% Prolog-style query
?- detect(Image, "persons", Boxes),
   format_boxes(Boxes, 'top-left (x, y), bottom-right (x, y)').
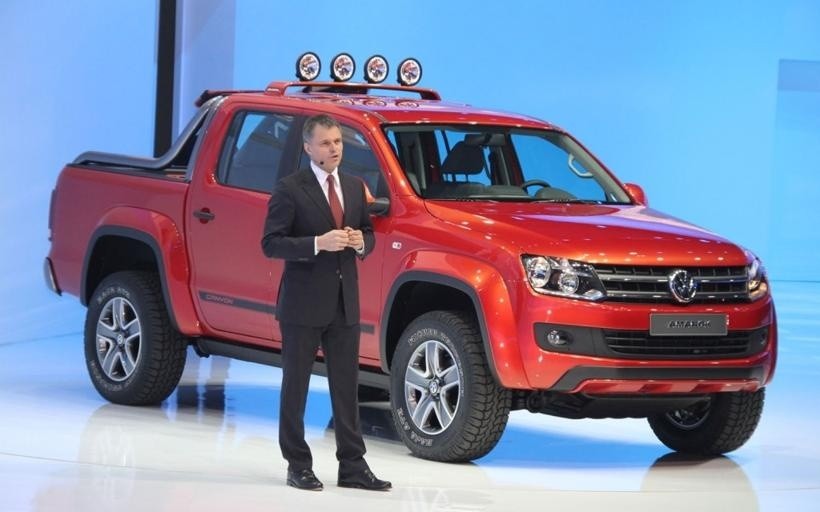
top-left (259, 112), bottom-right (396, 492)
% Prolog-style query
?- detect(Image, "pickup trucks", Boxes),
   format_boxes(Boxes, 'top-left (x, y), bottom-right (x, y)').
top-left (44, 50), bottom-right (782, 465)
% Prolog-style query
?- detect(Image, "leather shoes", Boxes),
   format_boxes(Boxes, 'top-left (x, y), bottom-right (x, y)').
top-left (287, 467), bottom-right (323, 491)
top-left (339, 467), bottom-right (391, 490)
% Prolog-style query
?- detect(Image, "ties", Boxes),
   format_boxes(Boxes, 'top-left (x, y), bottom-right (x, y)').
top-left (327, 174), bottom-right (344, 230)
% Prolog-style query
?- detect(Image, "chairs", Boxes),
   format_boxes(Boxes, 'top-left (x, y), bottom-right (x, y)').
top-left (422, 142), bottom-right (484, 199)
